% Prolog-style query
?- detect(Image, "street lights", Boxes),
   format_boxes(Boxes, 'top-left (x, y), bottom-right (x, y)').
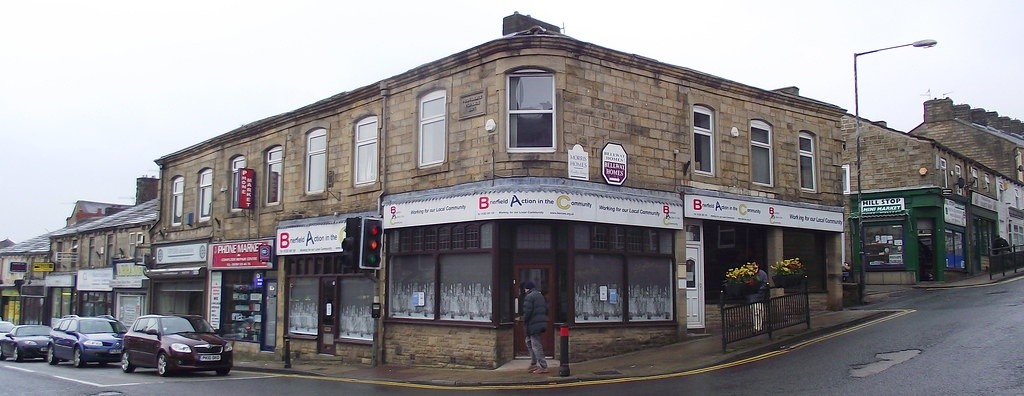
top-left (854, 39), bottom-right (939, 304)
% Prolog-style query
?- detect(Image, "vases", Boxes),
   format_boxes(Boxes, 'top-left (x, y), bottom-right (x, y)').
top-left (771, 273), bottom-right (803, 289)
top-left (724, 284), bottom-right (752, 300)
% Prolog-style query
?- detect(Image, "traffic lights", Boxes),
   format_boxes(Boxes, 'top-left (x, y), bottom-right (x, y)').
top-left (341, 218), bottom-right (361, 270)
top-left (359, 217), bottom-right (383, 270)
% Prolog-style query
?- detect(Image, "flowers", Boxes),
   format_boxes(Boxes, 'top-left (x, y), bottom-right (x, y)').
top-left (722, 262), bottom-right (760, 291)
top-left (768, 257), bottom-right (806, 275)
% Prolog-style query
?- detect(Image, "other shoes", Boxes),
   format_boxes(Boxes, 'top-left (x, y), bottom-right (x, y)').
top-left (528, 364), bottom-right (548, 373)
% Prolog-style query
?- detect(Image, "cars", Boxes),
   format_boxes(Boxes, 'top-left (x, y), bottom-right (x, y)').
top-left (0, 321), bottom-right (16, 338)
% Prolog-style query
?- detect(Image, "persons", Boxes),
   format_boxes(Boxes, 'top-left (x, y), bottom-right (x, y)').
top-left (992, 234), bottom-right (1011, 254)
top-left (749, 261), bottom-right (768, 333)
top-left (523, 281), bottom-right (548, 373)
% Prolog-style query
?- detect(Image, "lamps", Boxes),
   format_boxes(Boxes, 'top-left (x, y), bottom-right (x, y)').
top-left (954, 177), bottom-right (964, 187)
top-left (1017, 165), bottom-right (1024, 171)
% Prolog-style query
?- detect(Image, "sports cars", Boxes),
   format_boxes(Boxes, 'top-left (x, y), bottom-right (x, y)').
top-left (0, 325), bottom-right (53, 362)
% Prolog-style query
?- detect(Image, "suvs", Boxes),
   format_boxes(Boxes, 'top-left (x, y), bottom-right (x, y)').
top-left (121, 314), bottom-right (233, 377)
top-left (46, 314), bottom-right (128, 368)
